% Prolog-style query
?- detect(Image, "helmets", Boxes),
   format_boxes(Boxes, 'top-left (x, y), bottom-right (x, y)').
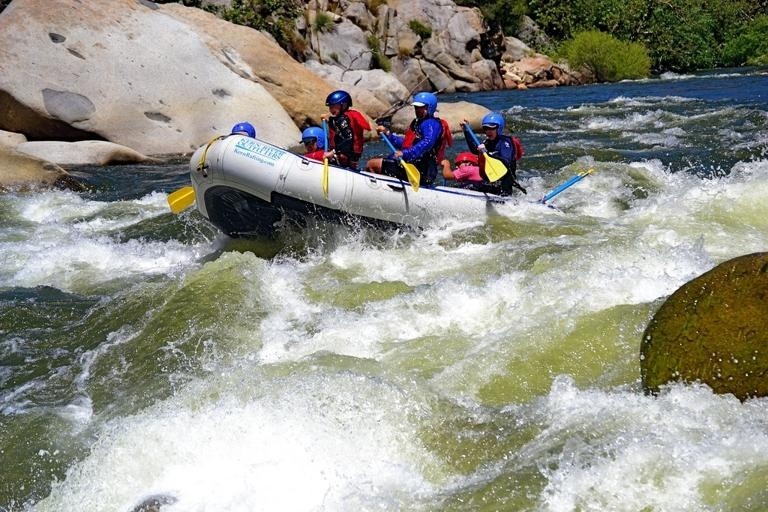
top-left (302, 126), bottom-right (328, 148)
top-left (411, 92), bottom-right (439, 115)
top-left (482, 111), bottom-right (506, 134)
top-left (232, 122), bottom-right (256, 139)
top-left (325, 89), bottom-right (353, 109)
top-left (454, 151), bottom-right (480, 166)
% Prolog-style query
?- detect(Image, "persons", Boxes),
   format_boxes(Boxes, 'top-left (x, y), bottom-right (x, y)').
top-left (367, 91), bottom-right (442, 186)
top-left (458, 112), bottom-right (523, 196)
top-left (319, 91), bottom-right (372, 170)
top-left (441, 153), bottom-right (483, 187)
top-left (298, 125), bottom-right (325, 161)
top-left (438, 119), bottom-right (453, 165)
top-left (233, 123), bottom-right (255, 140)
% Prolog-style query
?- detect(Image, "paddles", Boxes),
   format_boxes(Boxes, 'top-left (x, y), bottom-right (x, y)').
top-left (167, 186), bottom-right (195, 214)
top-left (322, 116), bottom-right (329, 199)
top-left (381, 131), bottom-right (421, 192)
top-left (464, 122), bottom-right (508, 183)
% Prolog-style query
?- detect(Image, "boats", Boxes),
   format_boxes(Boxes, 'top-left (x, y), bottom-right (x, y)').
top-left (189, 133), bottom-right (568, 248)
